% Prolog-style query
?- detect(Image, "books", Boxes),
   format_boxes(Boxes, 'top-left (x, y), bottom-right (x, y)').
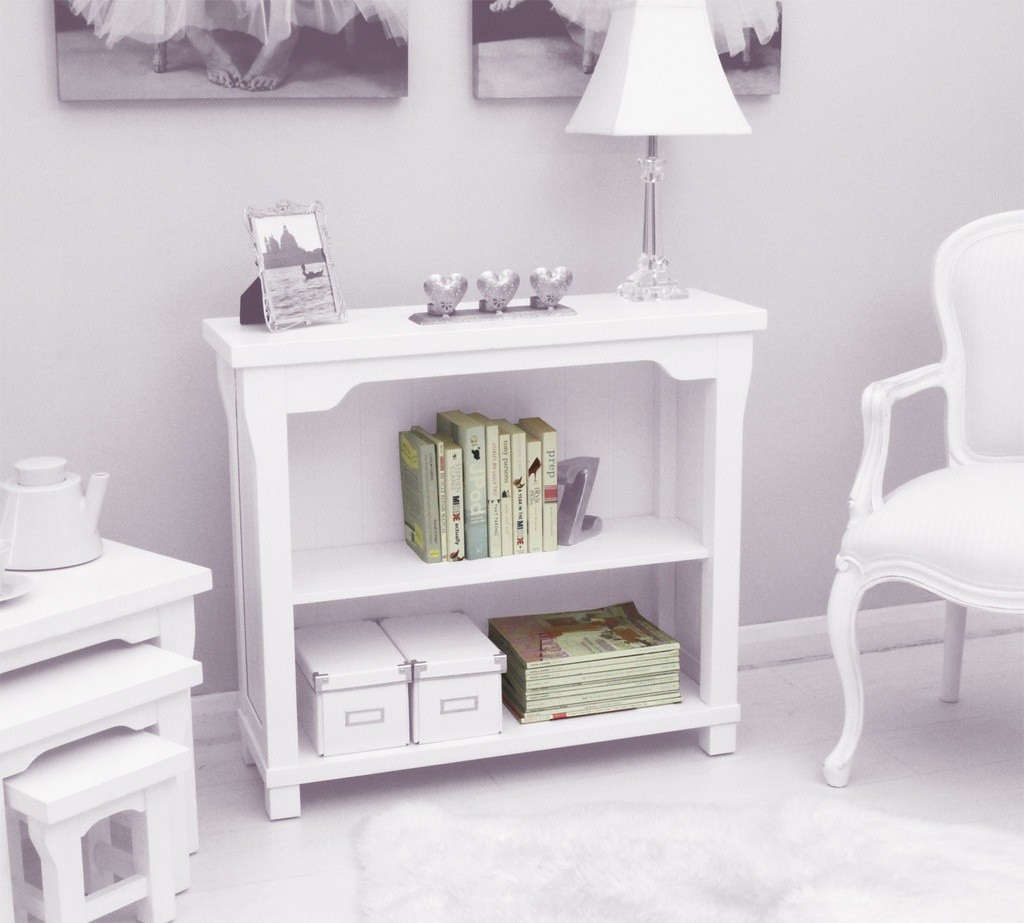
top-left (487, 600), bottom-right (683, 725)
top-left (410, 425), bottom-right (448, 563)
top-left (518, 416), bottom-right (558, 552)
top-left (436, 409), bottom-right (489, 561)
top-left (467, 411), bottom-right (503, 558)
top-left (513, 423), bottom-right (543, 552)
top-left (429, 433), bottom-right (465, 562)
top-left (491, 419), bottom-right (528, 554)
top-left (498, 427), bottom-right (514, 556)
top-left (398, 430), bottom-right (442, 564)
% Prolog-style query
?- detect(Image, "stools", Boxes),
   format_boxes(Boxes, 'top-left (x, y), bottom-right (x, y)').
top-left (4, 729), bottom-right (195, 923)
top-left (0, 641), bottom-right (207, 816)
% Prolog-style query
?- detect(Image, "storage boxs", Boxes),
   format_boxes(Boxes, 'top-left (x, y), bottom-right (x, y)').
top-left (294, 619), bottom-right (413, 757)
top-left (377, 610), bottom-right (509, 747)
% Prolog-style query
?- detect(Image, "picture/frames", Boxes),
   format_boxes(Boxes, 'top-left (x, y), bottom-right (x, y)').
top-left (470, 0), bottom-right (785, 102)
top-left (54, 1), bottom-right (410, 103)
top-left (238, 198), bottom-right (349, 333)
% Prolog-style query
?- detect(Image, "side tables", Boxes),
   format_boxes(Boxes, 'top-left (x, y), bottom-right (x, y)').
top-left (0, 535), bottom-right (213, 897)
top-left (200, 280), bottom-right (775, 822)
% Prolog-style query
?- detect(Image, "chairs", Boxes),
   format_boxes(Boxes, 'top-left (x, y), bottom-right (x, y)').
top-left (823, 208), bottom-right (1024, 791)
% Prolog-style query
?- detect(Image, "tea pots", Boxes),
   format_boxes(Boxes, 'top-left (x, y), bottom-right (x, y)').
top-left (1, 454), bottom-right (110, 574)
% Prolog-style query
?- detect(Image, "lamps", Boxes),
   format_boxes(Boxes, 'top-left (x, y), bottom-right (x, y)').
top-left (563, 0), bottom-right (757, 306)
